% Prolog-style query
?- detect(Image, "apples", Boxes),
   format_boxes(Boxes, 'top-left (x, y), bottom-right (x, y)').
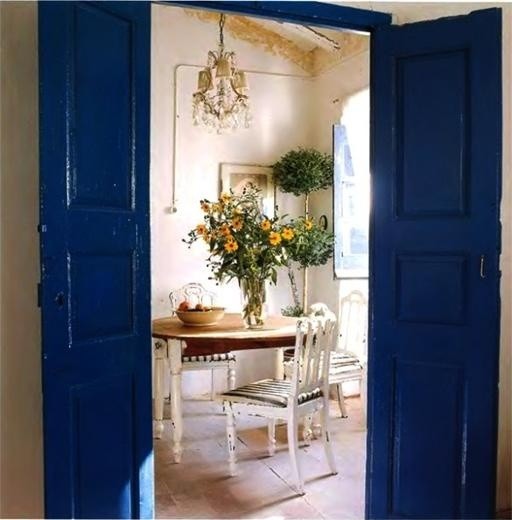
top-left (179, 301), bottom-right (190, 311)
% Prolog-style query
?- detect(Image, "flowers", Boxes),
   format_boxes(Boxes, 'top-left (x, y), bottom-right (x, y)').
top-left (181, 177), bottom-right (334, 283)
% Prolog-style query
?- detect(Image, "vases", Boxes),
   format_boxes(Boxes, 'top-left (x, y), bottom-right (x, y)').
top-left (240, 275), bottom-right (267, 329)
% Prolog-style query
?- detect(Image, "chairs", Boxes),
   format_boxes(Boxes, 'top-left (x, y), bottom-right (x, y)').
top-left (170, 281), bottom-right (237, 402)
top-left (220, 316), bottom-right (336, 495)
top-left (281, 302), bottom-right (362, 417)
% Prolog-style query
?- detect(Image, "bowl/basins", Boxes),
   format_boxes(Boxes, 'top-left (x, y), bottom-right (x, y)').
top-left (174, 307), bottom-right (225, 326)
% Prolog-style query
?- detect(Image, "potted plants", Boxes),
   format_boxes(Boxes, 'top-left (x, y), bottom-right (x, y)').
top-left (270, 149), bottom-right (335, 344)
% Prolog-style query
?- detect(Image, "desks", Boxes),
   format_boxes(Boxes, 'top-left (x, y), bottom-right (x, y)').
top-left (150, 312), bottom-right (334, 462)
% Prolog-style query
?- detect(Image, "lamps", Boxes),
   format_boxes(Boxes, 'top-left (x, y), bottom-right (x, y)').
top-left (192, 13), bottom-right (253, 134)
